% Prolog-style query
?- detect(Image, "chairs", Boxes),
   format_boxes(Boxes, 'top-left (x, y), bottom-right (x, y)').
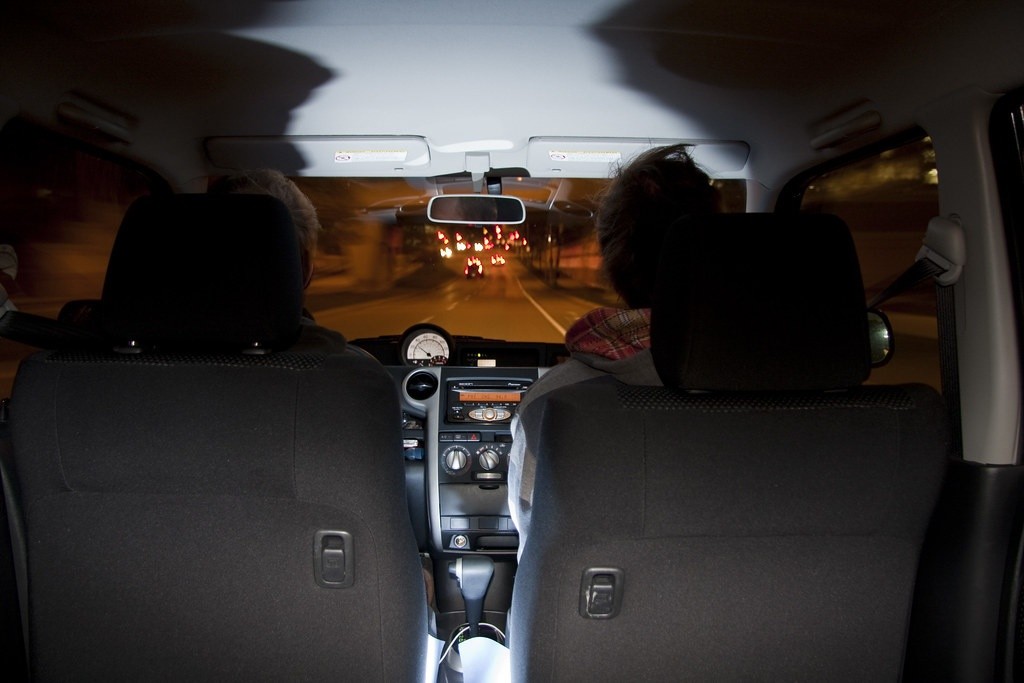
top-left (509, 215), bottom-right (945, 683)
top-left (0, 193), bottom-right (439, 683)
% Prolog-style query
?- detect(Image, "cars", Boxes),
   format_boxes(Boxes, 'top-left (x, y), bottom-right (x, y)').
top-left (465, 259), bottom-right (485, 279)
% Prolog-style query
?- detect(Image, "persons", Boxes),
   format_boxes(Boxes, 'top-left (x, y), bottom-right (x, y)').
top-left (504, 147), bottom-right (723, 647)
top-left (207, 168), bottom-right (319, 325)
top-left (430, 197), bottom-right (464, 221)
top-left (482, 206), bottom-right (497, 221)
